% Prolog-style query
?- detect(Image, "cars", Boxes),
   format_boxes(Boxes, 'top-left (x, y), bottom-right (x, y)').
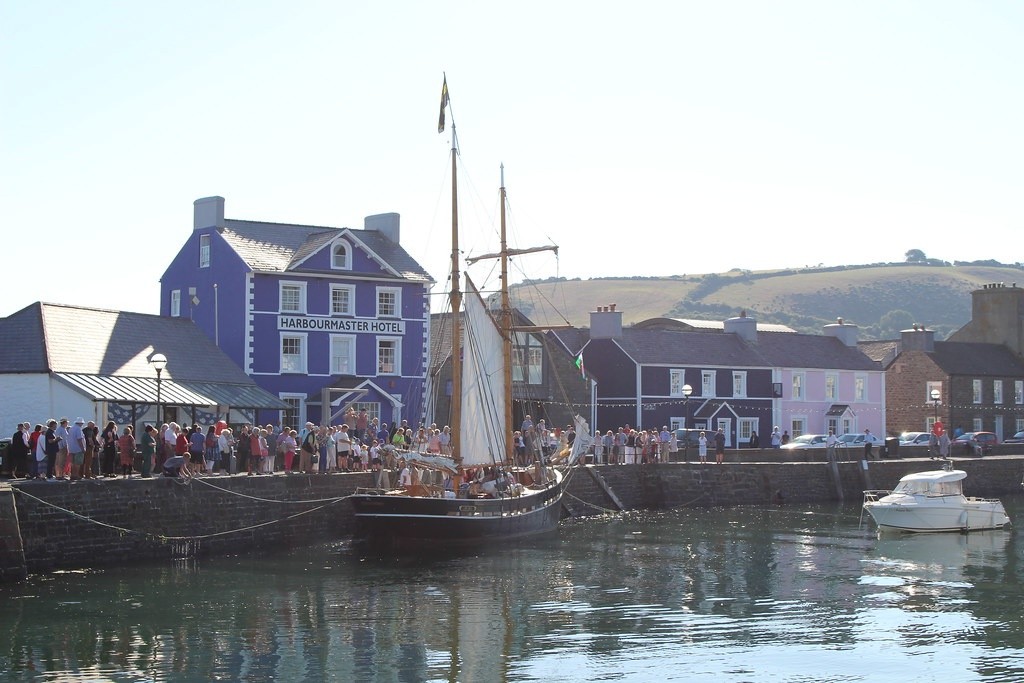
top-left (835, 433), bottom-right (884, 448)
top-left (951, 431), bottom-right (1000, 447)
top-left (897, 431), bottom-right (939, 447)
top-left (670, 427), bottom-right (717, 449)
top-left (779, 434), bottom-right (840, 450)
top-left (1005, 429), bottom-right (1024, 443)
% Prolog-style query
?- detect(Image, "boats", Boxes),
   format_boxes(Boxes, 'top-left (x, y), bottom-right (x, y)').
top-left (861, 459), bottom-right (1011, 533)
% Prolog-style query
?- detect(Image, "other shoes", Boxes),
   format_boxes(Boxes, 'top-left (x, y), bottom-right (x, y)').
top-left (142, 474), bottom-right (151, 478)
top-left (248, 473), bottom-right (252, 475)
top-left (128, 475), bottom-right (135, 479)
top-left (56, 477), bottom-right (67, 480)
top-left (70, 478), bottom-right (82, 480)
top-left (123, 475), bottom-right (126, 478)
top-left (256, 472), bottom-right (263, 475)
top-left (104, 473), bottom-right (117, 477)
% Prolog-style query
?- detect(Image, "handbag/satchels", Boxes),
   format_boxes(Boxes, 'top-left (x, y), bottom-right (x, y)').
top-left (128, 436), bottom-right (137, 458)
top-left (311, 454), bottom-right (318, 463)
top-left (262, 450), bottom-right (268, 456)
top-left (28, 439), bottom-right (34, 446)
top-left (211, 440), bottom-right (221, 459)
top-left (280, 441), bottom-right (288, 454)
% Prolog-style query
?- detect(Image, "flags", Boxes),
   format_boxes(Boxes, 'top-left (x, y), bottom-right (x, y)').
top-left (438, 81), bottom-right (449, 134)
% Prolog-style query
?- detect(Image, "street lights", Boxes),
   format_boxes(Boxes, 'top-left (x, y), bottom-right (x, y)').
top-left (150, 352), bottom-right (168, 474)
top-left (682, 384), bottom-right (692, 463)
top-left (931, 389), bottom-right (941, 447)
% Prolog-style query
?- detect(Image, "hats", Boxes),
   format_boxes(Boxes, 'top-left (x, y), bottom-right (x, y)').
top-left (39, 426), bottom-right (47, 432)
top-left (607, 430), bottom-right (613, 434)
top-left (595, 430), bottom-right (600, 434)
top-left (864, 429), bottom-right (870, 432)
top-left (306, 422), bottom-right (314, 426)
top-left (663, 426), bottom-right (668, 429)
top-left (58, 416), bottom-right (70, 422)
top-left (700, 432), bottom-right (705, 436)
top-left (829, 430), bottom-right (832, 433)
top-left (74, 417), bottom-right (85, 424)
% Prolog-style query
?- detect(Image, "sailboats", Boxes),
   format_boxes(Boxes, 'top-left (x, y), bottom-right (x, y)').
top-left (349, 64), bottom-right (603, 552)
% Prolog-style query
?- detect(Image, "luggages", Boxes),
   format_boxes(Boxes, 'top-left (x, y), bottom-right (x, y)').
top-left (228, 448), bottom-right (237, 475)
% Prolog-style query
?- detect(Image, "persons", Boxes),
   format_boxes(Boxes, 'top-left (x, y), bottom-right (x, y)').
top-left (749, 431), bottom-right (759, 448)
top-left (771, 426), bottom-right (781, 448)
top-left (594, 424), bottom-right (678, 464)
top-left (714, 428), bottom-right (725, 464)
top-left (399, 461), bottom-right (490, 491)
top-left (522, 415), bottom-right (532, 430)
top-left (953, 425), bottom-right (964, 438)
top-left (863, 429), bottom-right (874, 461)
top-left (826, 430), bottom-right (837, 461)
top-left (929, 429), bottom-right (950, 459)
top-left (512, 425), bottom-right (576, 467)
top-left (699, 432), bottom-right (708, 464)
top-left (10, 407), bottom-right (450, 481)
top-left (780, 431), bottom-right (789, 445)
top-left (538, 419), bottom-right (545, 430)
